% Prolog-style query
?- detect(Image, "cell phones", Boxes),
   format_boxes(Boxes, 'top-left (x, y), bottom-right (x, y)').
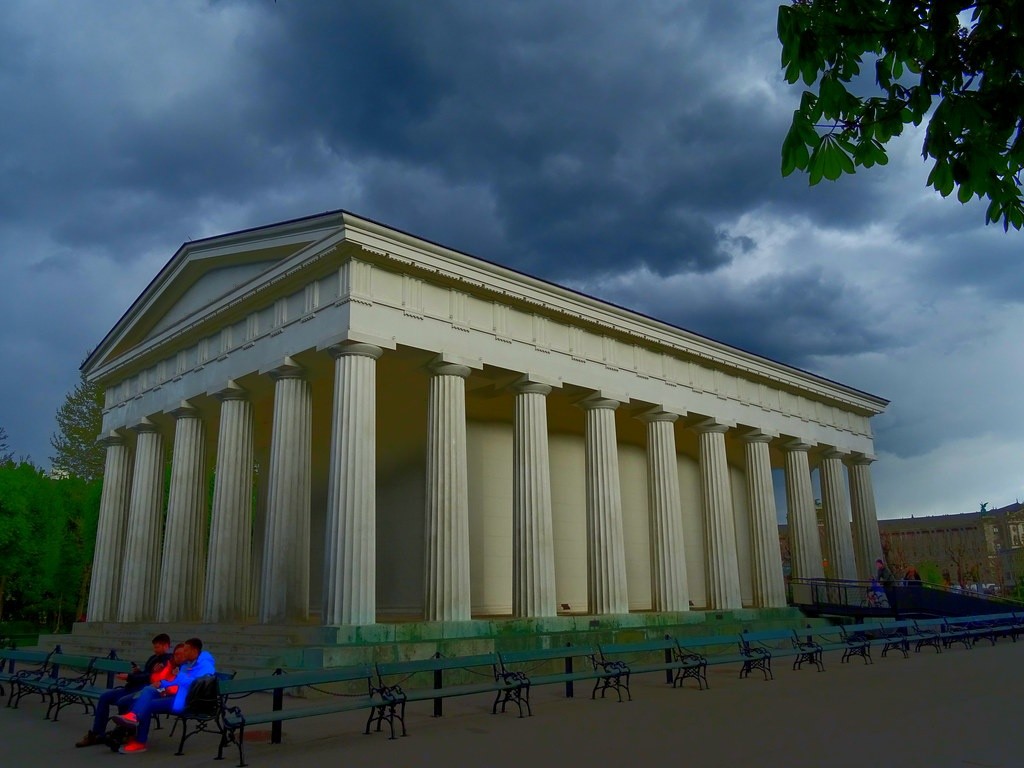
top-left (131, 662), bottom-right (136, 668)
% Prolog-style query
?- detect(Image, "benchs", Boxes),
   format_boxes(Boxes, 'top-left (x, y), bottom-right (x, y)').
top-left (944, 611), bottom-right (1024, 650)
top-left (674, 632), bottom-right (769, 690)
top-left (880, 620), bottom-right (940, 658)
top-left (792, 626), bottom-right (868, 672)
top-left (374, 653), bottom-right (525, 737)
top-left (497, 645), bottom-right (625, 717)
top-left (0, 642), bottom-right (58, 708)
top-left (50, 657), bottom-right (146, 722)
top-left (840, 623), bottom-right (907, 664)
top-left (738, 628), bottom-right (822, 681)
top-left (596, 639), bottom-right (704, 702)
top-left (914, 618), bottom-right (968, 654)
top-left (12, 645), bottom-right (115, 720)
top-left (151, 672), bottom-right (237, 756)
top-left (213, 664), bottom-right (400, 768)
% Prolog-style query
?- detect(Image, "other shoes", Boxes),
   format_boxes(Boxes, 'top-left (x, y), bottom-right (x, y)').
top-left (119, 741), bottom-right (147, 754)
top-left (75, 734), bottom-right (98, 747)
top-left (112, 711), bottom-right (138, 727)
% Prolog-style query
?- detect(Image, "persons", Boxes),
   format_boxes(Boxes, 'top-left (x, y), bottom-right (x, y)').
top-left (902, 566), bottom-right (924, 588)
top-left (76, 633), bottom-right (216, 754)
top-left (865, 558), bottom-right (896, 604)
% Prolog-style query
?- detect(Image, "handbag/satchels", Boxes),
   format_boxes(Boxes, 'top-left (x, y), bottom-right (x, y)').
top-left (189, 673), bottom-right (218, 716)
